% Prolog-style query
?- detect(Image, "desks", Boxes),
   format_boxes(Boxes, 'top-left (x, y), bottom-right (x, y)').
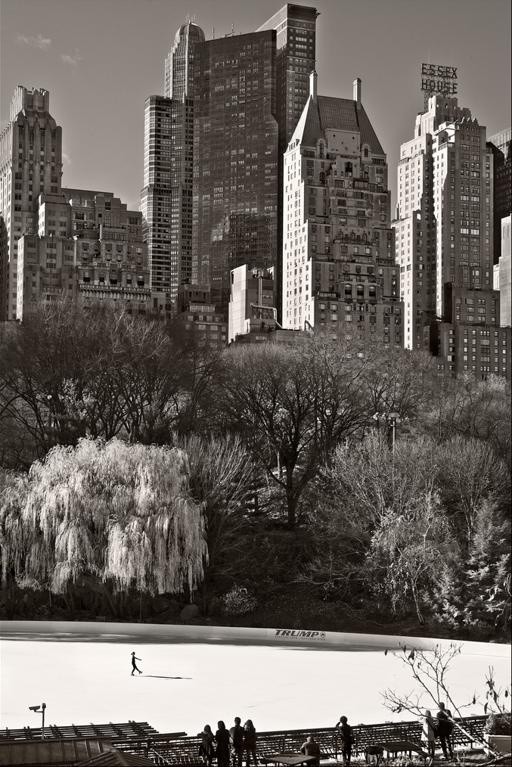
top-left (382, 740), bottom-right (419, 761)
top-left (260, 753), bottom-right (317, 767)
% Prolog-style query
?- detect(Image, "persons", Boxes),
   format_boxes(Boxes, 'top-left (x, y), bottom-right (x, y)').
top-left (420, 710), bottom-right (436, 756)
top-left (300, 736), bottom-right (320, 767)
top-left (243, 719), bottom-right (257, 767)
top-left (131, 652), bottom-right (143, 675)
top-left (338, 716), bottom-right (352, 762)
top-left (215, 721), bottom-right (231, 767)
top-left (435, 702), bottom-right (453, 759)
top-left (230, 717), bottom-right (244, 767)
top-left (197, 725), bottom-right (215, 767)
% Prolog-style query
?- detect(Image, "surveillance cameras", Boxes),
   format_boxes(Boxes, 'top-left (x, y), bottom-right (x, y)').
top-left (29, 705), bottom-right (40, 711)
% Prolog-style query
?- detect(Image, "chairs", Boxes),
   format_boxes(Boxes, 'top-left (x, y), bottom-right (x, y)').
top-left (363, 745), bottom-right (384, 767)
top-left (305, 744), bottom-right (320, 767)
